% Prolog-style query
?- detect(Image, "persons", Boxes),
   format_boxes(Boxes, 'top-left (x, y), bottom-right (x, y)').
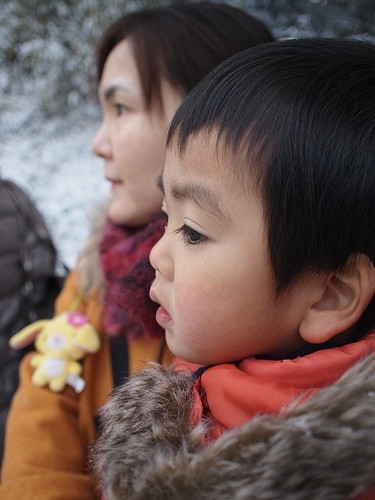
top-left (0, 0), bottom-right (279, 500)
top-left (88, 37), bottom-right (374, 500)
